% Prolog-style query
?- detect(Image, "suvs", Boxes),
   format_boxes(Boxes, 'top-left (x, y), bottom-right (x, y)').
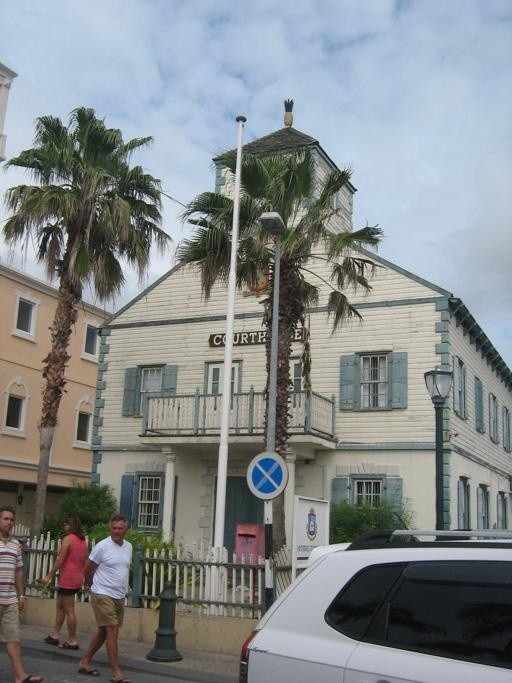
top-left (236, 524), bottom-right (512, 682)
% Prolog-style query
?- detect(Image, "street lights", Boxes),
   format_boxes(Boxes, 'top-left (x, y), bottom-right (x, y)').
top-left (419, 359), bottom-right (456, 548)
top-left (252, 207), bottom-right (291, 622)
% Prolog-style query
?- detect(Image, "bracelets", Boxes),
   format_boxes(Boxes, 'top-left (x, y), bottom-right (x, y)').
top-left (18, 595), bottom-right (25, 598)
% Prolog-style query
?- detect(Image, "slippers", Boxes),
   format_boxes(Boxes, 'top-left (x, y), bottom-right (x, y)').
top-left (12, 673), bottom-right (45, 683)
top-left (43, 634), bottom-right (59, 646)
top-left (109, 675), bottom-right (132, 683)
top-left (58, 640), bottom-right (80, 650)
top-left (77, 662), bottom-right (101, 677)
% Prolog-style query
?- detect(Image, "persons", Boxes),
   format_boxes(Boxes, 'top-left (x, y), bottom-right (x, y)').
top-left (0, 506), bottom-right (45, 682)
top-left (40, 513), bottom-right (87, 648)
top-left (78, 514), bottom-right (133, 682)
